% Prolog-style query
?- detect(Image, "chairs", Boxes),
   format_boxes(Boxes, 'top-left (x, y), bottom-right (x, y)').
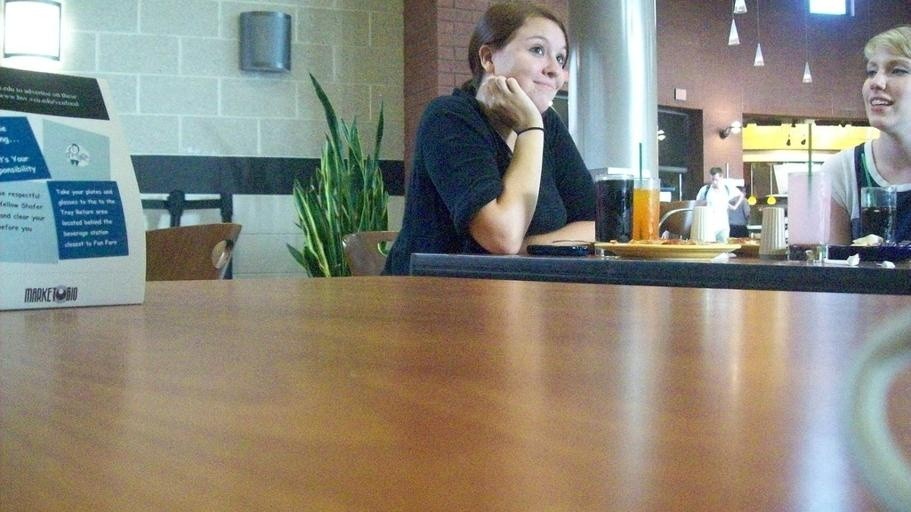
top-left (339, 228), bottom-right (400, 274)
top-left (145, 223), bottom-right (243, 279)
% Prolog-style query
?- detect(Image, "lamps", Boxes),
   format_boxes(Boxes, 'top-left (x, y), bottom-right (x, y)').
top-left (725, 1), bottom-right (815, 84)
top-left (2, 1), bottom-right (64, 64)
top-left (238, 11), bottom-right (291, 75)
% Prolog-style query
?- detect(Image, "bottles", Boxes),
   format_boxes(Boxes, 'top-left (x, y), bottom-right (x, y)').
top-left (688, 205), bottom-right (717, 243)
top-left (757, 206), bottom-right (788, 261)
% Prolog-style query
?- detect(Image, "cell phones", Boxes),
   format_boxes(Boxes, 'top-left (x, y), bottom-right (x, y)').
top-left (527, 244), bottom-right (590, 256)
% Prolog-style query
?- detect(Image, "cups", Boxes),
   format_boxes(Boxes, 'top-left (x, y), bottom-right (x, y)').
top-left (860, 186), bottom-right (898, 247)
top-left (630, 176), bottom-right (661, 244)
top-left (593, 173), bottom-right (632, 245)
top-left (787, 164), bottom-right (831, 264)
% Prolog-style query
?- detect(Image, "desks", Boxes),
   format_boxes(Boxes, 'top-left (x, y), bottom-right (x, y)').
top-left (0, 272), bottom-right (906, 511)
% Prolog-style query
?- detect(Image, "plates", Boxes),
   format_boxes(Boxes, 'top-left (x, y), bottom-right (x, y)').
top-left (590, 239), bottom-right (742, 263)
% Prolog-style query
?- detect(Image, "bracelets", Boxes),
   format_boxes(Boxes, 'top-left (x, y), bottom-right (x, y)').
top-left (516, 126), bottom-right (545, 133)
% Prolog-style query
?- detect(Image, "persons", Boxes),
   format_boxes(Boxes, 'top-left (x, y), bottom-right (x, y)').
top-left (820, 24), bottom-right (911, 247)
top-left (728, 185), bottom-right (752, 238)
top-left (696, 165), bottom-right (744, 245)
top-left (376, 0), bottom-right (596, 276)
